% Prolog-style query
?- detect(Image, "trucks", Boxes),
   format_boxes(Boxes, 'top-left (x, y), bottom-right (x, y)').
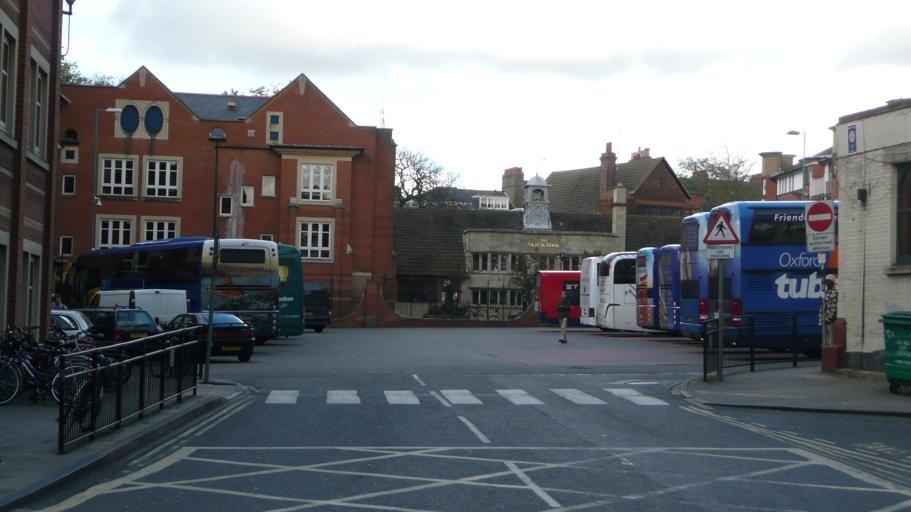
top-left (89, 289), bottom-right (188, 326)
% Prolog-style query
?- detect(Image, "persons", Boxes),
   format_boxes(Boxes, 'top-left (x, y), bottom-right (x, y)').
top-left (557, 291), bottom-right (572, 343)
top-left (51, 294), bottom-right (69, 310)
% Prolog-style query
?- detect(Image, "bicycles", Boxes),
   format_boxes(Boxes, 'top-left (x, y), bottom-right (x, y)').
top-left (149, 336), bottom-right (190, 379)
top-left (0, 325), bottom-right (131, 438)
top-left (463, 302), bottom-right (488, 321)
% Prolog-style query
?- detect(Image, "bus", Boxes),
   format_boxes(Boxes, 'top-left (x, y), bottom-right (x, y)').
top-left (61, 235), bottom-right (278, 342)
top-left (679, 200), bottom-right (838, 355)
top-left (579, 243), bottom-right (680, 338)
top-left (277, 245), bottom-right (305, 338)
top-left (533, 271), bottom-right (581, 326)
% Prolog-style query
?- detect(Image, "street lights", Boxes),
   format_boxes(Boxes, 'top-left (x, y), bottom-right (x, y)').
top-left (91, 107), bottom-right (122, 251)
top-left (787, 129), bottom-right (808, 199)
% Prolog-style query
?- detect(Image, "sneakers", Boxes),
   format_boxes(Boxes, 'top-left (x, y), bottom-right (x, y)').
top-left (558, 339), bottom-right (567, 343)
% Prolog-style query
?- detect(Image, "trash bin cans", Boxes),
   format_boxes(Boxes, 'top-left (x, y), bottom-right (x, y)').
top-left (878, 310), bottom-right (911, 394)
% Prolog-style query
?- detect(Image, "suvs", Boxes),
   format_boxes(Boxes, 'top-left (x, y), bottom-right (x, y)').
top-left (48, 306), bottom-right (162, 357)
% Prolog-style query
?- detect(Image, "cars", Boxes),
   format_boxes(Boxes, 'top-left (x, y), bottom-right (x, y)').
top-left (165, 312), bottom-right (254, 363)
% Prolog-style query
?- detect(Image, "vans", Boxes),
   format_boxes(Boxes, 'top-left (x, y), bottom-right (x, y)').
top-left (304, 283), bottom-right (332, 333)
